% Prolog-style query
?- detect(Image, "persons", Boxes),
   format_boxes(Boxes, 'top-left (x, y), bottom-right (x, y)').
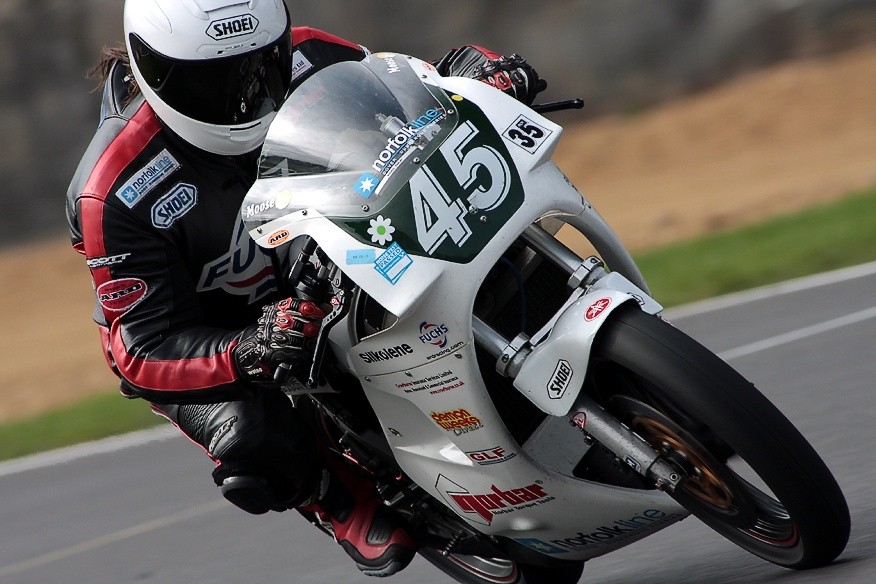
top-left (65, 0), bottom-right (539, 576)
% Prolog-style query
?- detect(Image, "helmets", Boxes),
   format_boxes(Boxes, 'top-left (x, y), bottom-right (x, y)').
top-left (124, 0), bottom-right (293, 161)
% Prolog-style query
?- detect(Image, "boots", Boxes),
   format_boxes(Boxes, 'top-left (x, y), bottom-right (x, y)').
top-left (293, 459), bottom-right (418, 577)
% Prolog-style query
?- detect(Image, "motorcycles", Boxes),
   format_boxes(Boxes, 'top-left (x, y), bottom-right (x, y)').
top-left (239, 50), bottom-right (852, 584)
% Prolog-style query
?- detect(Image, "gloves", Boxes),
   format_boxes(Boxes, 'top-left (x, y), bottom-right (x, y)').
top-left (469, 53), bottom-right (548, 107)
top-left (233, 297), bottom-right (334, 390)
